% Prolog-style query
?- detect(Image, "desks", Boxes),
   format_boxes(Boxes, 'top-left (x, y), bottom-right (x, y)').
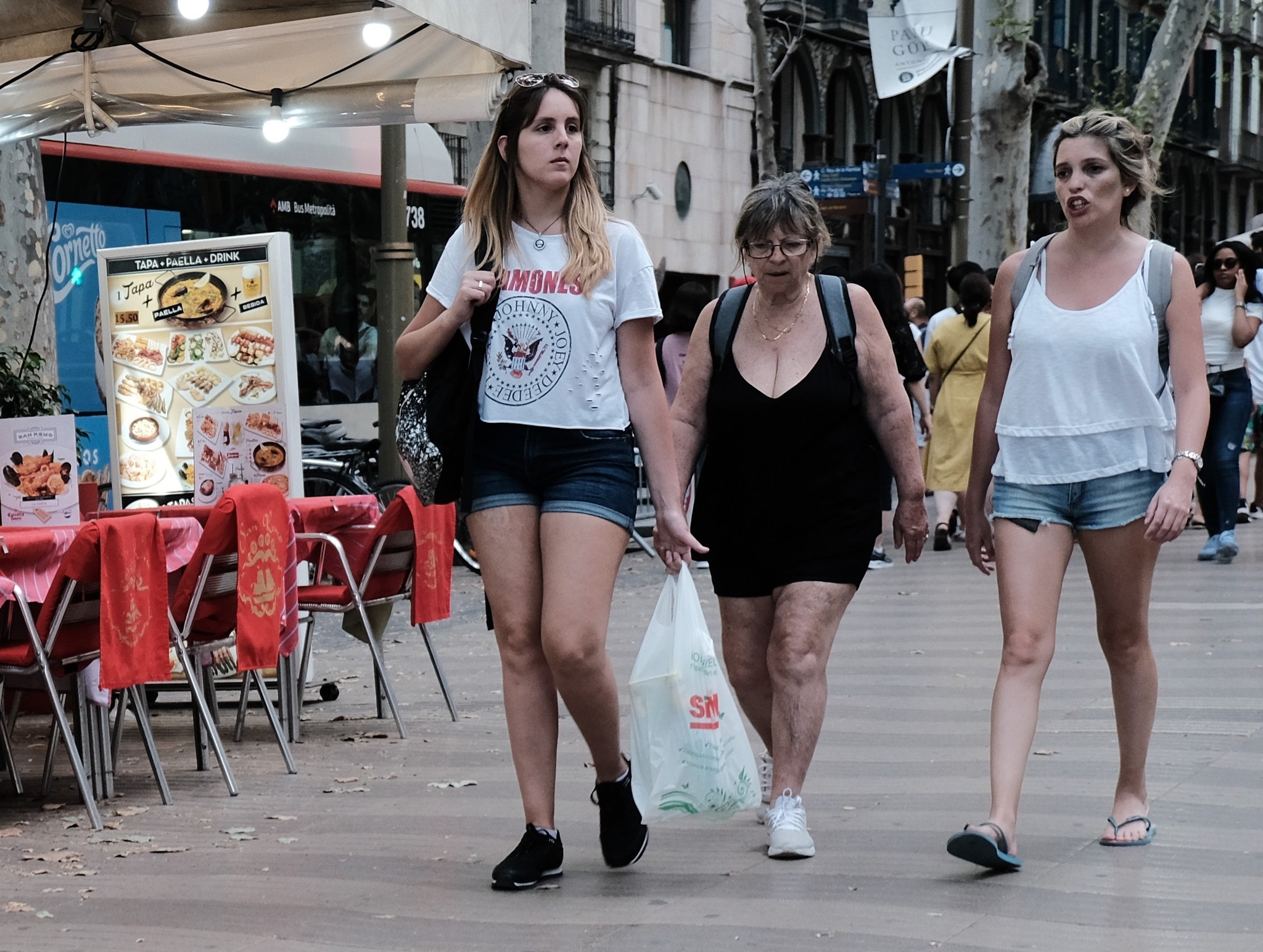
top-left (0, 517), bottom-right (203, 797)
top-left (88, 493), bottom-right (378, 743)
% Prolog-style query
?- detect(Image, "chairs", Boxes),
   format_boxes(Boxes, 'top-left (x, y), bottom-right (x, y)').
top-left (294, 485), bottom-right (459, 741)
top-left (0, 513), bottom-right (174, 831)
top-left (166, 483), bottom-right (297, 795)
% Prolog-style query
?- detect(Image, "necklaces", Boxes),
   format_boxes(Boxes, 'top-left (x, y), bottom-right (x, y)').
top-left (756, 271), bottom-right (812, 334)
top-left (518, 210), bottom-right (565, 249)
top-left (753, 288), bottom-right (808, 339)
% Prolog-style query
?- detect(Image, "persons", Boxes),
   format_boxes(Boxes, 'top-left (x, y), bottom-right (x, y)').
top-left (947, 102), bottom-right (1211, 868)
top-left (647, 171), bottom-right (929, 861)
top-left (385, 74), bottom-right (711, 889)
top-left (286, 273), bottom-right (380, 406)
top-left (922, 260), bottom-right (989, 373)
top-left (646, 229), bottom-right (1262, 570)
top-left (927, 275), bottom-right (997, 552)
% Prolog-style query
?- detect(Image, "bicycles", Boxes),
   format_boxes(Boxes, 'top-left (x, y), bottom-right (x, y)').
top-left (299, 411), bottom-right (482, 575)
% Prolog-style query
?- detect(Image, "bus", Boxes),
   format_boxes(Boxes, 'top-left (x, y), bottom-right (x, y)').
top-left (34, 119), bottom-right (468, 509)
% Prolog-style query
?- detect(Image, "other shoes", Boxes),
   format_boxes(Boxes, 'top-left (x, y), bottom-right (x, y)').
top-left (952, 525), bottom-right (967, 542)
top-left (1197, 534), bottom-right (1221, 560)
top-left (1236, 498), bottom-right (1252, 523)
top-left (1248, 504), bottom-right (1263, 519)
top-left (696, 560), bottom-right (709, 569)
top-left (1216, 530), bottom-right (1239, 564)
top-left (868, 549), bottom-right (893, 569)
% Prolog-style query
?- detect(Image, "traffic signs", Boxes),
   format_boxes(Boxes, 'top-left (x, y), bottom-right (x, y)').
top-left (808, 181), bottom-right (864, 199)
top-left (888, 161), bottom-right (966, 179)
top-left (800, 164), bottom-right (863, 186)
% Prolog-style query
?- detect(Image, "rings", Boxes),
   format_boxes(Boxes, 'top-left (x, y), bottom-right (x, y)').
top-left (478, 280), bottom-right (483, 290)
top-left (926, 534), bottom-right (929, 539)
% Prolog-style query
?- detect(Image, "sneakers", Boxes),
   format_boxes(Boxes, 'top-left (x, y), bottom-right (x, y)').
top-left (590, 750), bottom-right (649, 868)
top-left (755, 748), bottom-right (774, 824)
top-left (764, 786), bottom-right (815, 858)
top-left (491, 822), bottom-right (564, 891)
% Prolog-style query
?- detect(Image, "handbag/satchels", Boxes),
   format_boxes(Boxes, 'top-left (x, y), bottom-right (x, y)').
top-left (627, 558), bottom-right (762, 825)
top-left (425, 214), bottom-right (502, 459)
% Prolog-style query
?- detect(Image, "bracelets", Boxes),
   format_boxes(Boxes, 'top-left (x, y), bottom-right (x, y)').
top-left (1235, 301), bottom-right (1247, 310)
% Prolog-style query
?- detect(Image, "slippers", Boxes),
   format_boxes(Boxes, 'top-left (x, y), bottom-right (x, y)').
top-left (1099, 814), bottom-right (1157, 846)
top-left (947, 821), bottom-right (1021, 868)
top-left (1191, 522), bottom-right (1205, 529)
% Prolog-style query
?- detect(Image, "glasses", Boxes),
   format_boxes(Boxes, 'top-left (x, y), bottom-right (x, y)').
top-left (1212, 257), bottom-right (1239, 270)
top-left (506, 73), bottom-right (579, 113)
top-left (743, 237), bottom-right (813, 259)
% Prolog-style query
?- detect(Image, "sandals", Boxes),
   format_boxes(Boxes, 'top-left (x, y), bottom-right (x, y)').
top-left (933, 522), bottom-right (953, 551)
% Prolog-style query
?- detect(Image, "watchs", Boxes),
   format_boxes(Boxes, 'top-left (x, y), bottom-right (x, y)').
top-left (1172, 449), bottom-right (1203, 473)
